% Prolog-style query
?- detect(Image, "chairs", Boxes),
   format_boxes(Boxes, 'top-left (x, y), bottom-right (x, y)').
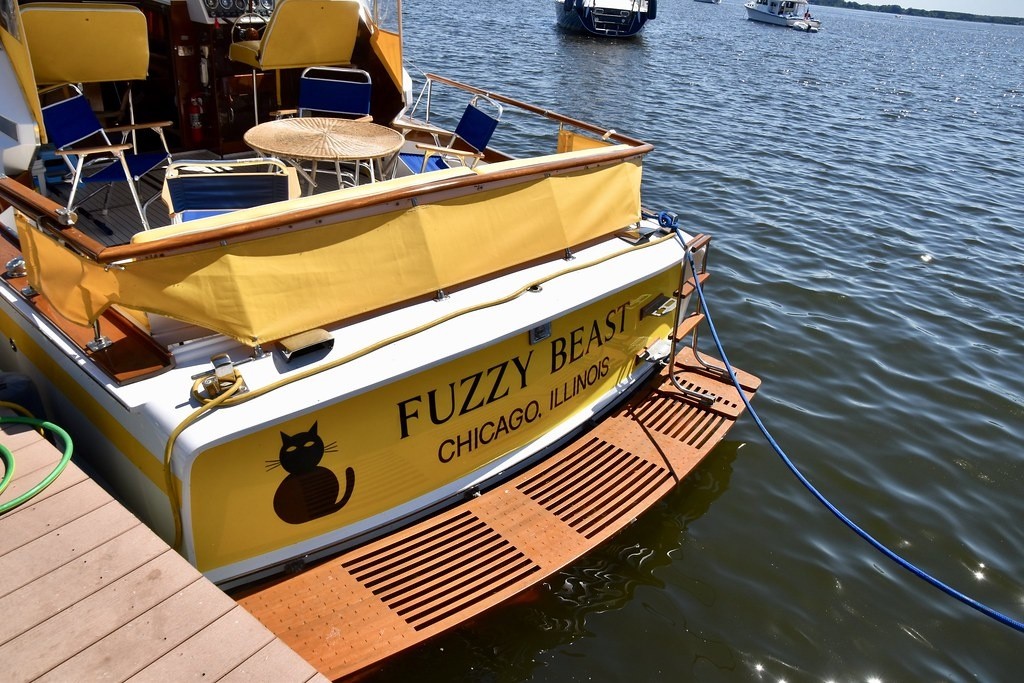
top-left (229, 0), bottom-right (360, 128)
top-left (37, 82), bottom-right (174, 231)
top-left (276, 66), bottom-right (372, 196)
top-left (161, 157), bottom-right (302, 225)
top-left (20, 2), bottom-right (149, 155)
top-left (375, 94), bottom-right (503, 182)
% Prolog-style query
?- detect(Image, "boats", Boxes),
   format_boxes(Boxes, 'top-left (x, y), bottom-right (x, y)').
top-left (0, 1), bottom-right (760, 683)
top-left (742, 0), bottom-right (823, 34)
top-left (554, 0), bottom-right (657, 39)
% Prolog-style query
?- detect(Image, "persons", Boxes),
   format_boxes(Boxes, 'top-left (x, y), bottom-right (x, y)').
top-left (806, 10), bottom-right (810, 18)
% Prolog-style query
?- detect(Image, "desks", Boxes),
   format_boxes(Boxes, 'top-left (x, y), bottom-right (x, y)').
top-left (243, 117), bottom-right (405, 196)
